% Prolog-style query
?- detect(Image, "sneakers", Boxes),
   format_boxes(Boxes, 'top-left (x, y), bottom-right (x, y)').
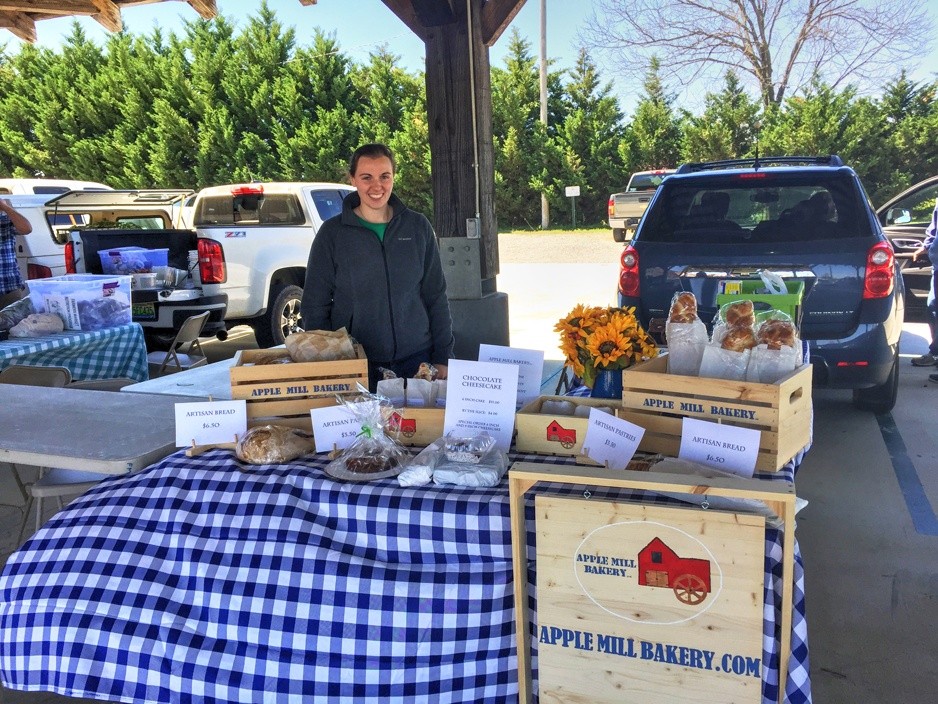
top-left (928, 374), bottom-right (938, 383)
top-left (911, 353), bottom-right (938, 365)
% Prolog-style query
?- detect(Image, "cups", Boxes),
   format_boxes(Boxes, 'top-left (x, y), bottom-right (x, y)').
top-left (187, 249), bottom-right (202, 287)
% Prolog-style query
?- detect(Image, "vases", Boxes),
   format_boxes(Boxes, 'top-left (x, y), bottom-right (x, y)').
top-left (593, 369), bottom-right (621, 399)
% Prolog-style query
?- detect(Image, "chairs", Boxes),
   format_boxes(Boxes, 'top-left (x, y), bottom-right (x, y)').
top-left (672, 204), bottom-right (742, 244)
top-left (31, 375), bottom-right (140, 535)
top-left (759, 202), bottom-right (812, 238)
top-left (147, 309), bottom-right (214, 377)
top-left (0, 364), bottom-right (71, 552)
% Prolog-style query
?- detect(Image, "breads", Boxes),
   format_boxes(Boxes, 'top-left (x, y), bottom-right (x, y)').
top-left (236, 424), bottom-right (316, 464)
top-left (415, 362), bottom-right (433, 381)
top-left (668, 292), bottom-right (796, 350)
top-left (346, 446), bottom-right (399, 473)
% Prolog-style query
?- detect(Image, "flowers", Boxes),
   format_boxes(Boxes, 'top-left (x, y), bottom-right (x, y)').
top-left (553, 299), bottom-right (661, 386)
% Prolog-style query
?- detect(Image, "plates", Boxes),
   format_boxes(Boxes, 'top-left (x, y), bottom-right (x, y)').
top-left (326, 454), bottom-right (410, 480)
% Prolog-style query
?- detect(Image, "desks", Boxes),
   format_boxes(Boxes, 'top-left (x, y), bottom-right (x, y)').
top-left (123, 338), bottom-right (287, 393)
top-left (0, 321), bottom-right (150, 385)
top-left (0, 379), bottom-right (234, 478)
top-left (0, 429), bottom-right (815, 704)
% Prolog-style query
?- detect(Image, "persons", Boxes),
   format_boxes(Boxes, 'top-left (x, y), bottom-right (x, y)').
top-left (0, 196), bottom-right (34, 312)
top-left (912, 197), bottom-right (938, 385)
top-left (299, 144), bottom-right (456, 396)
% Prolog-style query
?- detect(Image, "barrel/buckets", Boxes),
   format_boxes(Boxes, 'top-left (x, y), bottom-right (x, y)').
top-left (129, 273), bottom-right (157, 290)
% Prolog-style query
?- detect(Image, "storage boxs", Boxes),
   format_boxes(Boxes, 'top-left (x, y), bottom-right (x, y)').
top-left (95, 245), bottom-right (170, 275)
top-left (379, 398), bottom-right (446, 447)
top-left (515, 391), bottom-right (620, 461)
top-left (621, 350), bottom-right (814, 472)
top-left (23, 272), bottom-right (134, 331)
top-left (223, 345), bottom-right (370, 435)
